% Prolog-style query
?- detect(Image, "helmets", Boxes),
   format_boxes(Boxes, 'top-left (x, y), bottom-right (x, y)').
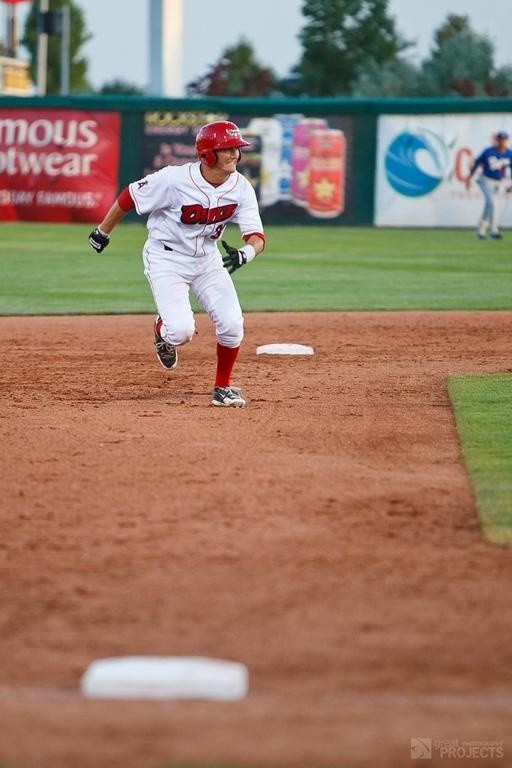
top-left (195, 120), bottom-right (251, 169)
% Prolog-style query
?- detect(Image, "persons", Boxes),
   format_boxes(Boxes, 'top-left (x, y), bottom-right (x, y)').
top-left (462, 128), bottom-right (512, 240)
top-left (86, 117), bottom-right (268, 407)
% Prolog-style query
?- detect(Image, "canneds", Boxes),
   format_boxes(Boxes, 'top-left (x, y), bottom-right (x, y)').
top-left (238, 114), bottom-right (347, 219)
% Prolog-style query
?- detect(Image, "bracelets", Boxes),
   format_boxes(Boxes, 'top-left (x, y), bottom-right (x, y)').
top-left (238, 243), bottom-right (256, 264)
top-left (96, 223), bottom-right (112, 239)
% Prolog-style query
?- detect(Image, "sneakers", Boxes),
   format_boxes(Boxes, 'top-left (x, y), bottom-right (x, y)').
top-left (475, 231), bottom-right (502, 240)
top-left (212, 384), bottom-right (246, 408)
top-left (154, 315), bottom-right (178, 371)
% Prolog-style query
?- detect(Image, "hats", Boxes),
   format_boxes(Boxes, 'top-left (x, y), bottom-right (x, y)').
top-left (495, 131), bottom-right (509, 141)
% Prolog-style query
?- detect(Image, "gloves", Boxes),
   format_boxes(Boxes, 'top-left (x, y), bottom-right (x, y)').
top-left (220, 241), bottom-right (256, 275)
top-left (87, 228), bottom-right (111, 255)
